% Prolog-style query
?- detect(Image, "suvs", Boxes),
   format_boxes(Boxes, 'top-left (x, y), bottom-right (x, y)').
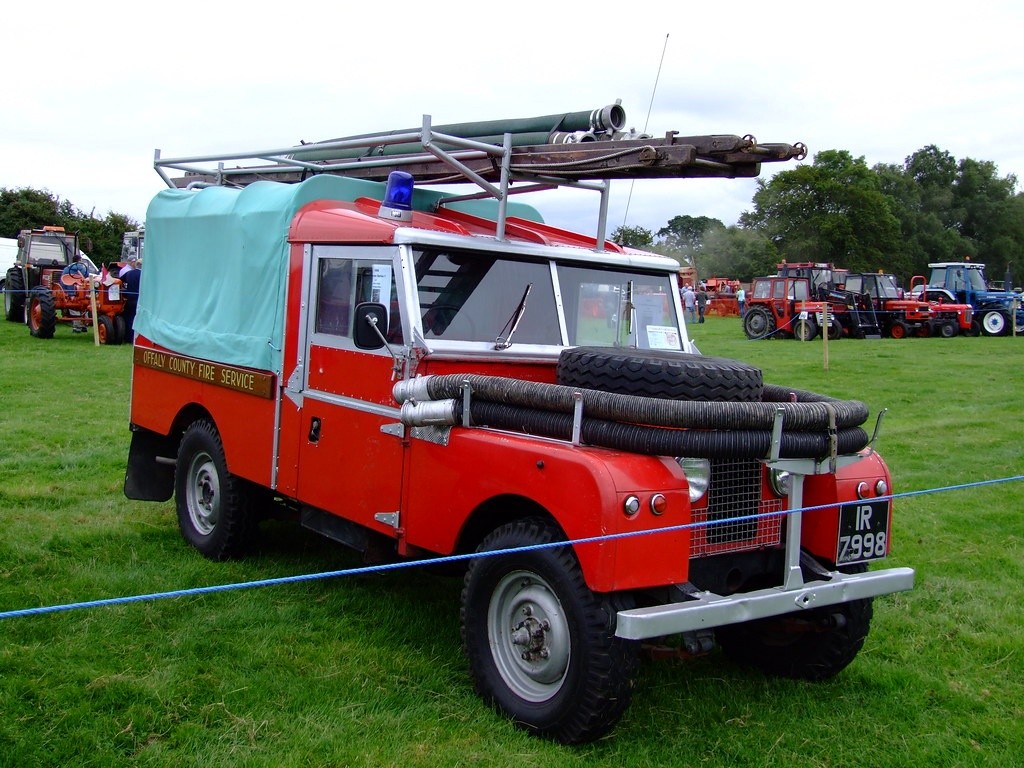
top-left (125, 100), bottom-right (914, 746)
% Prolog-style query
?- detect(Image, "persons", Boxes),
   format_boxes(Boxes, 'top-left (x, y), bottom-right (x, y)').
top-left (695, 286), bottom-right (709, 324)
top-left (683, 286), bottom-right (697, 325)
top-left (721, 283), bottom-right (731, 295)
top-left (680, 281), bottom-right (689, 297)
top-left (116, 255), bottom-right (137, 289)
top-left (118, 258), bottom-right (142, 343)
top-left (60, 254), bottom-right (90, 334)
top-left (735, 287), bottom-right (746, 318)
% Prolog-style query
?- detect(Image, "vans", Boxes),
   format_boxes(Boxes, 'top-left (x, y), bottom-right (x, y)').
top-left (0, 237), bottom-right (99, 294)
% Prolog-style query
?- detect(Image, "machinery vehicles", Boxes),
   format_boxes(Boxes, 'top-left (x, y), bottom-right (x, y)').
top-left (740, 276), bottom-right (843, 341)
top-left (777, 259), bottom-right (883, 340)
top-left (5, 227), bottom-right (82, 322)
top-left (25, 262), bottom-right (129, 346)
top-left (843, 273), bottom-right (933, 339)
top-left (927, 263), bottom-right (1024, 337)
top-left (903, 288), bottom-right (981, 339)
